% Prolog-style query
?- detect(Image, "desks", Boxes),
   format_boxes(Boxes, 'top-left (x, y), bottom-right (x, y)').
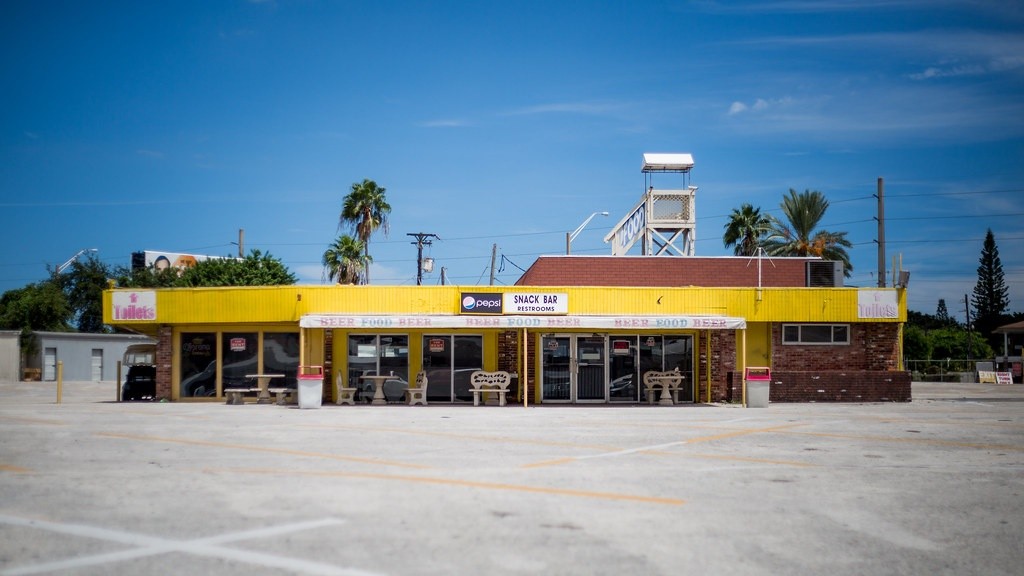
top-left (647, 376), bottom-right (686, 406)
top-left (245, 374), bottom-right (286, 404)
top-left (360, 375), bottom-right (400, 404)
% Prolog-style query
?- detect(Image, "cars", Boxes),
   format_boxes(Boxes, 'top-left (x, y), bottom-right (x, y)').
top-left (608, 373), bottom-right (635, 397)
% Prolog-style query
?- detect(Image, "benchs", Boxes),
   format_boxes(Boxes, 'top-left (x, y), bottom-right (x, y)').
top-left (402, 370), bottom-right (429, 405)
top-left (270, 388), bottom-right (300, 406)
top-left (224, 387), bottom-right (250, 406)
top-left (335, 369), bottom-right (357, 406)
top-left (642, 371), bottom-right (684, 405)
top-left (249, 388), bottom-right (288, 402)
top-left (469, 370), bottom-right (511, 407)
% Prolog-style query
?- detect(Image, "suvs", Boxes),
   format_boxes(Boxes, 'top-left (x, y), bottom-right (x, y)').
top-left (121, 363), bottom-right (156, 401)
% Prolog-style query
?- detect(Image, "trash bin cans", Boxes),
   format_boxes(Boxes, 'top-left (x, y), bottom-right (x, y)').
top-left (296, 365), bottom-right (324, 408)
top-left (745, 366), bottom-right (772, 408)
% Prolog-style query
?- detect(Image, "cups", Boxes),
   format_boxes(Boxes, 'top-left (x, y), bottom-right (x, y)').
top-left (675, 367), bottom-right (679, 376)
top-left (390, 371), bottom-right (394, 376)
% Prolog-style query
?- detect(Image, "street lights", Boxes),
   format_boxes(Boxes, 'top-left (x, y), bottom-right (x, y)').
top-left (566, 211), bottom-right (609, 255)
